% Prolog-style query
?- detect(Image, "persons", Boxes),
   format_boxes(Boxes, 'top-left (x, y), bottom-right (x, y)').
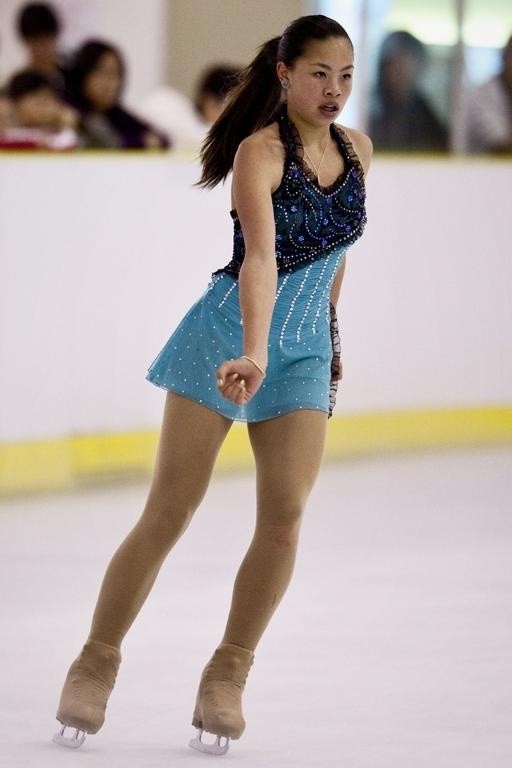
top-left (55, 16), bottom-right (374, 740)
top-left (1, 1), bottom-right (245, 160)
top-left (365, 31), bottom-right (511, 154)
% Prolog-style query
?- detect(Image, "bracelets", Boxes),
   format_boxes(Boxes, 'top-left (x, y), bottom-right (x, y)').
top-left (240, 354), bottom-right (269, 380)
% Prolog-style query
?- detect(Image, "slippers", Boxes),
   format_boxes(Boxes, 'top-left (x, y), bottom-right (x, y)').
top-left (55, 637), bottom-right (124, 734)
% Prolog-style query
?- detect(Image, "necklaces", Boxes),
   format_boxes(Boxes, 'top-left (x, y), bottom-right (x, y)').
top-left (304, 136), bottom-right (331, 184)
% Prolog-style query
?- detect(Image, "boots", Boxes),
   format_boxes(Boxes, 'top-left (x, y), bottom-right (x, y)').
top-left (191, 643), bottom-right (256, 741)
